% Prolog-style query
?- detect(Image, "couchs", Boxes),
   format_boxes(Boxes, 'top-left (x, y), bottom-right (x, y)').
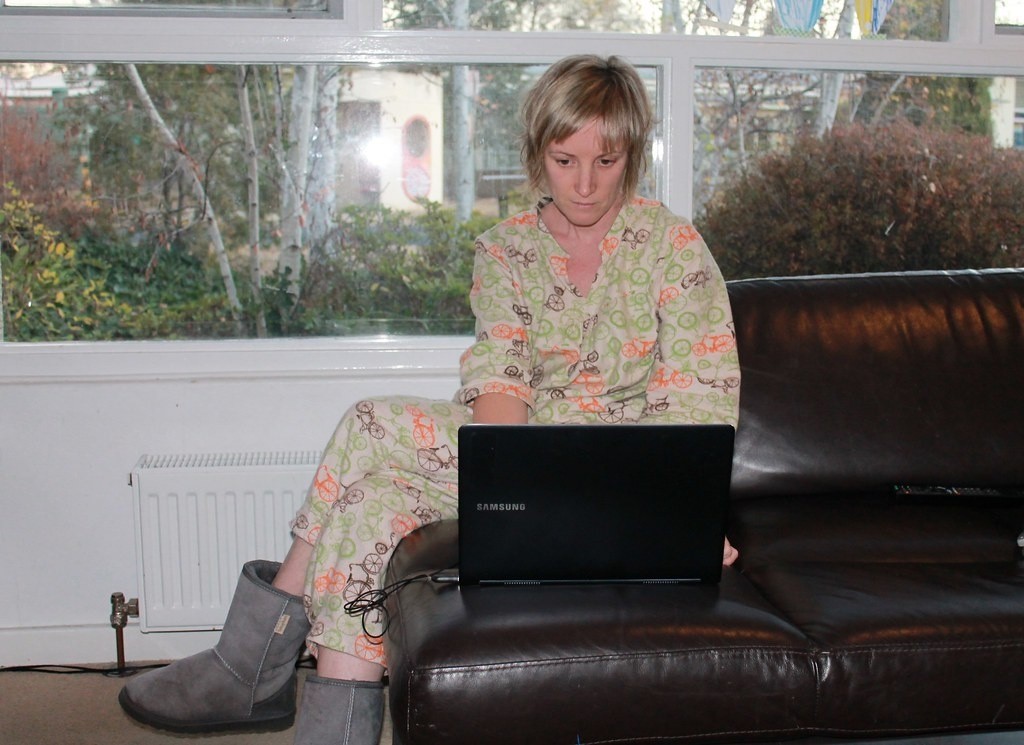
top-left (382, 268), bottom-right (1024, 745)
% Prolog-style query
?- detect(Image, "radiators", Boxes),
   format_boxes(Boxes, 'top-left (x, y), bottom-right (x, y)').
top-left (131, 451), bottom-right (325, 635)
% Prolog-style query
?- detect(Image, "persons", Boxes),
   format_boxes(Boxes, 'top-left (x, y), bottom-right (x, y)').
top-left (117, 54), bottom-right (739, 745)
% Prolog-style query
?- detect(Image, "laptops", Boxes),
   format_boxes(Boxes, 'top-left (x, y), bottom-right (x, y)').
top-left (457, 424), bottom-right (736, 588)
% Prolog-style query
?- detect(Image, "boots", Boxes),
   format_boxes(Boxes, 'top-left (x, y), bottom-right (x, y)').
top-left (116, 561), bottom-right (314, 739)
top-left (293, 672), bottom-right (384, 745)
